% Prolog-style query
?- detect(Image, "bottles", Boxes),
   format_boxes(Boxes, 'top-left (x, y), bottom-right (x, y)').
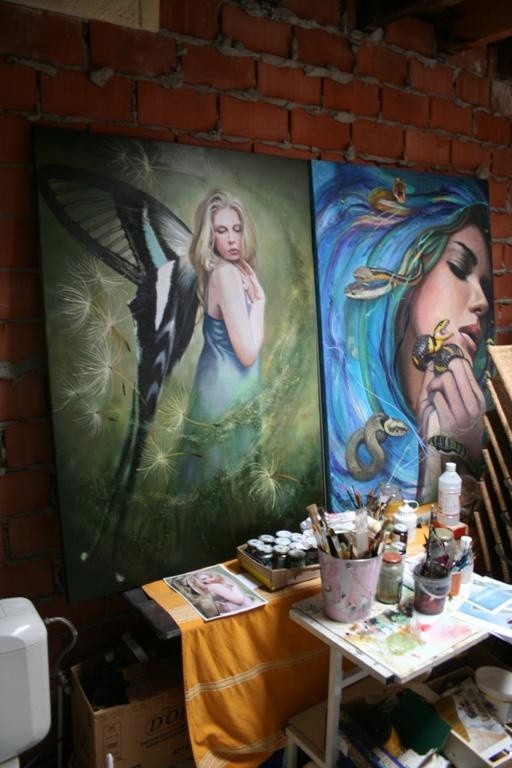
top-left (246, 530), bottom-right (318, 568)
top-left (378, 481), bottom-right (417, 604)
top-left (433, 462), bottom-right (474, 583)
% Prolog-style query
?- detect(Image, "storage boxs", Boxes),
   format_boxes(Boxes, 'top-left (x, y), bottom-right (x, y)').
top-left (70, 644), bottom-right (193, 768)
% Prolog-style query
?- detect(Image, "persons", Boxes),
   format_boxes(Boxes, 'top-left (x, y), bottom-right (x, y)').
top-left (316, 163), bottom-right (491, 512)
top-left (187, 570), bottom-right (246, 615)
top-left (166, 190), bottom-right (266, 494)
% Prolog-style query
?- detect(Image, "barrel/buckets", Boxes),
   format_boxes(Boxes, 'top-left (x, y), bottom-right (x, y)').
top-left (413, 563), bottom-right (449, 615)
top-left (316, 533), bottom-right (383, 622)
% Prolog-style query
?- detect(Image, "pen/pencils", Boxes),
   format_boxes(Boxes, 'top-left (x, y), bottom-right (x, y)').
top-left (306, 483), bottom-right (396, 559)
top-left (449, 536), bottom-right (479, 572)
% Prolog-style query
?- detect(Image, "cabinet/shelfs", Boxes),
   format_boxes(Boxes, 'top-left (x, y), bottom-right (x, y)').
top-left (284, 567), bottom-right (512, 767)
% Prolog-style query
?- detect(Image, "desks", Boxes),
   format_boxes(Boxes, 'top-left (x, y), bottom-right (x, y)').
top-left (123, 560), bottom-right (350, 768)
